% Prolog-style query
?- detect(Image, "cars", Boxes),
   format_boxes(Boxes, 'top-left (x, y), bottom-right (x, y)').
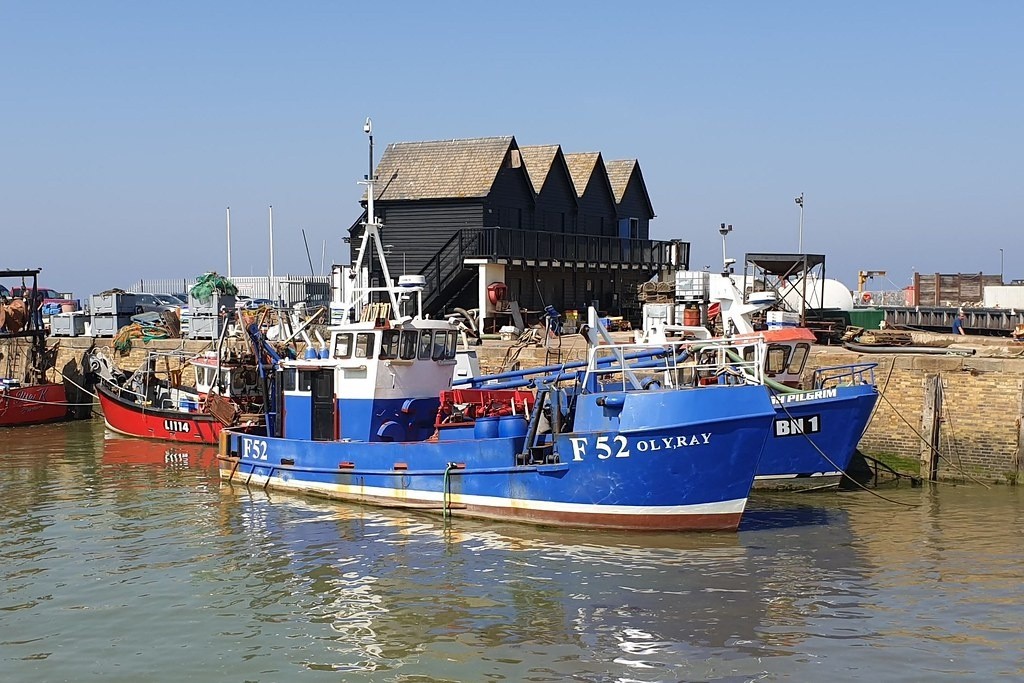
top-left (218, 295), bottom-right (278, 319)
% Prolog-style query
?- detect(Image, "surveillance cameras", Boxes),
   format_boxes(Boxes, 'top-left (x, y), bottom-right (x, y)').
top-left (364, 125), bottom-right (370, 133)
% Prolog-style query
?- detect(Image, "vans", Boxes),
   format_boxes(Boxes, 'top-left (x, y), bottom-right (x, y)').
top-left (78, 291), bottom-right (190, 338)
top-left (0, 283), bottom-right (77, 314)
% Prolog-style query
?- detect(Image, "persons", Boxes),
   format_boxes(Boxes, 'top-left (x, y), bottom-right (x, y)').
top-left (952, 312), bottom-right (966, 335)
top-left (32, 293), bottom-right (45, 329)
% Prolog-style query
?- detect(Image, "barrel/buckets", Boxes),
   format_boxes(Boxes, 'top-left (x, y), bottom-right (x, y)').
top-left (305, 347), bottom-right (316, 361)
top-left (317, 346), bottom-right (328, 360)
top-left (473, 417), bottom-right (500, 439)
top-left (498, 415), bottom-right (527, 438)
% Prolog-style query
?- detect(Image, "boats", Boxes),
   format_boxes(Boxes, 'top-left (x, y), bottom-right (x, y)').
top-left (79, 330), bottom-right (274, 447)
top-left (431, 340), bottom-right (880, 536)
top-left (0, 266), bottom-right (71, 429)
top-left (215, 117), bottom-right (779, 536)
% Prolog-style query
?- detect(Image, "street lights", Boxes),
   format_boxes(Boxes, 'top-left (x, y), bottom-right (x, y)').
top-left (1000, 248), bottom-right (1003, 283)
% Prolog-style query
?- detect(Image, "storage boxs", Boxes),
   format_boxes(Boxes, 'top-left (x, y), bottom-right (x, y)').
top-left (499, 326), bottom-right (519, 341)
top-left (561, 310), bottom-right (578, 333)
top-left (767, 311), bottom-right (799, 330)
top-left (597, 319), bottom-right (611, 332)
